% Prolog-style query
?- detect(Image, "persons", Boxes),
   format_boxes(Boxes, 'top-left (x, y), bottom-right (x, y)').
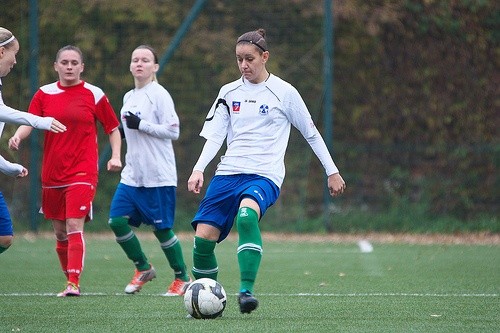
top-left (107, 45), bottom-right (193, 297)
top-left (8, 44), bottom-right (123, 298)
top-left (188, 28), bottom-right (346, 319)
top-left (0, 27), bottom-right (67, 254)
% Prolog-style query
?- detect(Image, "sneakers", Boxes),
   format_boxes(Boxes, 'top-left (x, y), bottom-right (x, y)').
top-left (65, 282), bottom-right (80, 298)
top-left (124, 262), bottom-right (158, 295)
top-left (163, 276), bottom-right (193, 297)
top-left (237, 290), bottom-right (259, 314)
top-left (56, 289), bottom-right (65, 297)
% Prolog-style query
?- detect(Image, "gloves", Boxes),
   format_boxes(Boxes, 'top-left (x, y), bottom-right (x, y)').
top-left (124, 111), bottom-right (142, 130)
top-left (118, 128), bottom-right (125, 138)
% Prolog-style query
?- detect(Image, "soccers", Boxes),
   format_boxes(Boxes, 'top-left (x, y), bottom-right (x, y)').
top-left (184, 277), bottom-right (227, 320)
